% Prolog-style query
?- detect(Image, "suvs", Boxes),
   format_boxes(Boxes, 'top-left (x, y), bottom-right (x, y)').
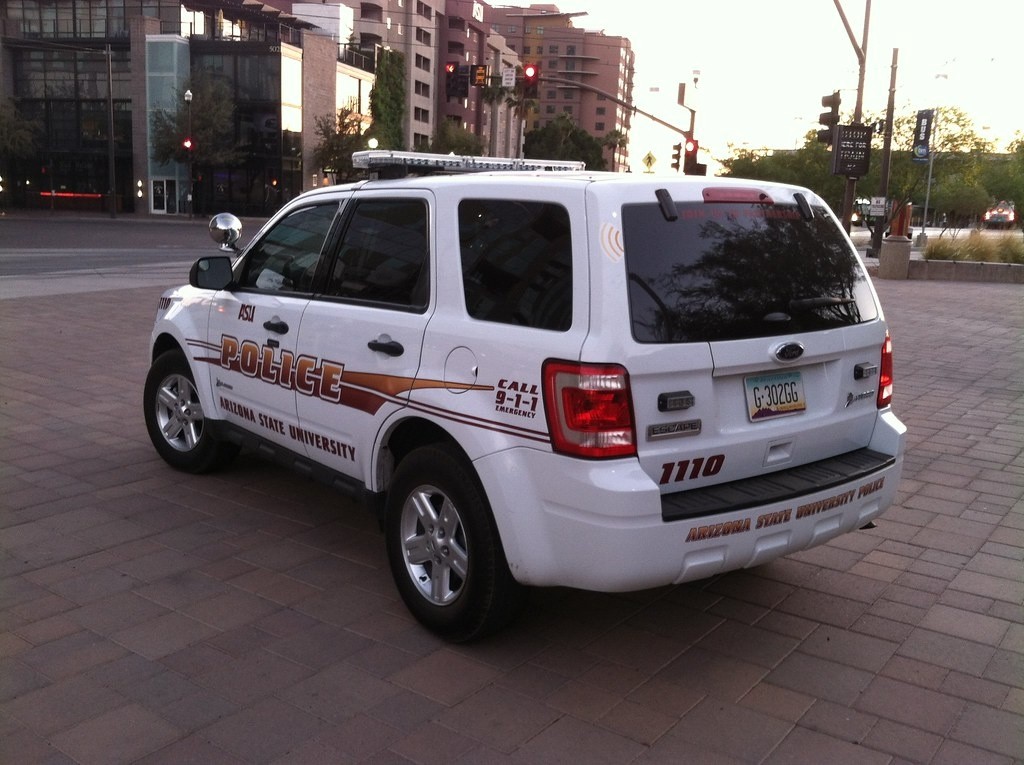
top-left (142, 150), bottom-right (910, 646)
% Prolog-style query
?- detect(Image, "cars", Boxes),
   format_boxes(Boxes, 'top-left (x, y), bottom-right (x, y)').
top-left (984, 203), bottom-right (1015, 227)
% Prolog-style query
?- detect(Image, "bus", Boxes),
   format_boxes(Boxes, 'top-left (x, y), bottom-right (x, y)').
top-left (852, 198), bottom-right (878, 226)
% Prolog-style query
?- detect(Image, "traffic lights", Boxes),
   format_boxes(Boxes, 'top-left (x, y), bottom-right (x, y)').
top-left (524, 64), bottom-right (539, 98)
top-left (671, 142), bottom-right (682, 171)
top-left (818, 90), bottom-right (841, 145)
top-left (445, 62), bottom-right (467, 97)
top-left (684, 139), bottom-right (699, 174)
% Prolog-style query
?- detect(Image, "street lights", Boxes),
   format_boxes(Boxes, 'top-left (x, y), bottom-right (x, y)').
top-left (183, 88), bottom-right (194, 216)
